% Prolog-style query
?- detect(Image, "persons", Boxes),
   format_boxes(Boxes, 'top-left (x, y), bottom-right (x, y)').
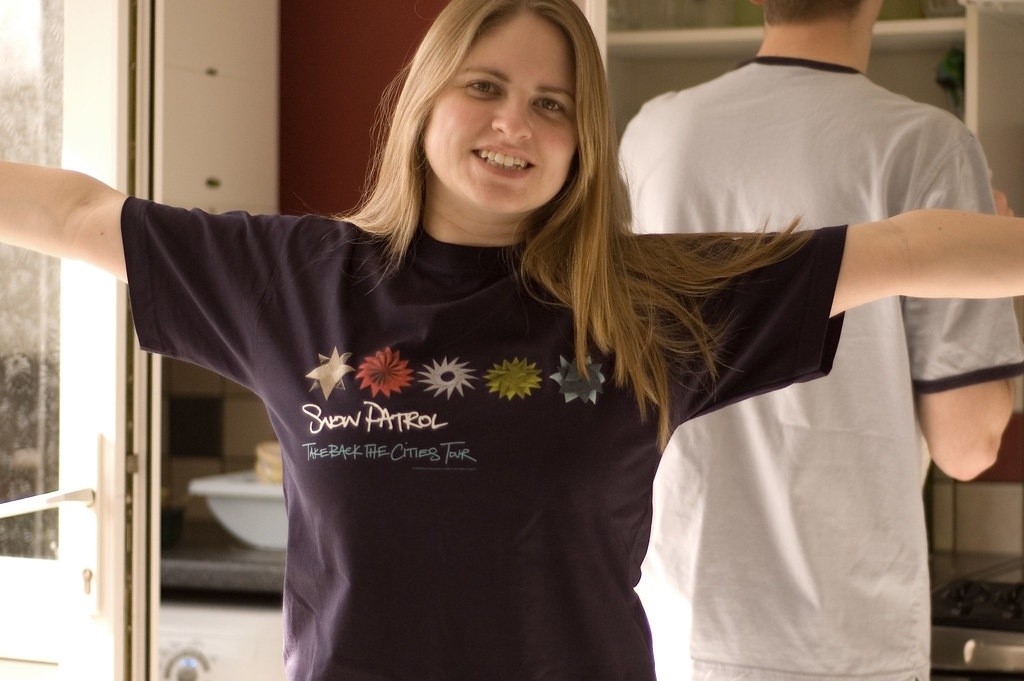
top-left (624, 3), bottom-right (1022, 680)
top-left (0, 0), bottom-right (1024, 681)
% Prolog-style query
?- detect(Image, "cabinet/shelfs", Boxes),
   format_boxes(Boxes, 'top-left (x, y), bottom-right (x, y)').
top-left (584, 0), bottom-right (1024, 179)
